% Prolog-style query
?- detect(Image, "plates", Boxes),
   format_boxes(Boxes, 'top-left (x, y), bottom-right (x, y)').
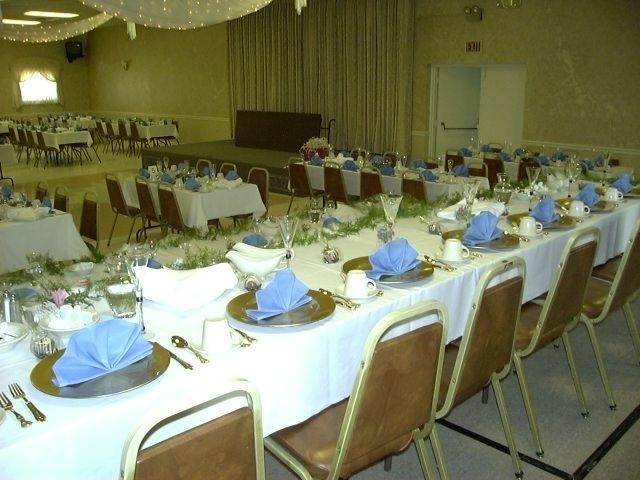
top-left (568, 213), bottom-right (592, 221)
top-left (436, 257), bottom-right (471, 266)
top-left (29, 340), bottom-right (171, 399)
top-left (227, 288), bottom-right (336, 329)
top-left (606, 201), bottom-right (626, 205)
top-left (341, 291), bottom-right (379, 304)
top-left (517, 232), bottom-right (544, 240)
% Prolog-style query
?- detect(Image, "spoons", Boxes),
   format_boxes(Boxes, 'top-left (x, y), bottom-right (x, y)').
top-left (424, 255), bottom-right (458, 271)
top-left (171, 336), bottom-right (210, 364)
top-left (503, 230), bottom-right (531, 242)
top-left (319, 288), bottom-right (361, 308)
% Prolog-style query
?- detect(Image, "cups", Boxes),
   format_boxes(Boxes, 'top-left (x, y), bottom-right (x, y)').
top-left (603, 187), bottom-right (624, 201)
top-left (569, 200), bottom-right (590, 217)
top-left (105, 282), bottom-right (137, 320)
top-left (517, 216), bottom-right (543, 235)
top-left (442, 239), bottom-right (470, 261)
top-left (343, 269), bottom-right (376, 297)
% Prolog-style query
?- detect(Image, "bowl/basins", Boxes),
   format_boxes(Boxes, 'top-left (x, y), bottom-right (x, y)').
top-left (0, 322), bottom-right (30, 353)
top-left (66, 262), bottom-right (93, 276)
top-left (38, 312), bottom-right (100, 347)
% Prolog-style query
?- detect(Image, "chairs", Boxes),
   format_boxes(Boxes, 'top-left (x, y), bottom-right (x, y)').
top-left (105, 122), bottom-right (125, 156)
top-left (36, 129), bottom-right (59, 170)
top-left (0, 177), bottom-right (15, 196)
top-left (35, 179), bottom-right (50, 204)
top-left (232, 166), bottom-right (270, 230)
top-left (359, 165), bottom-right (386, 201)
top-left (286, 156), bottom-right (326, 216)
top-left (128, 121), bottom-right (145, 159)
top-left (217, 159), bottom-right (240, 179)
top-left (70, 127), bottom-right (102, 167)
top-left (119, 382), bottom-right (266, 480)
top-left (0, 113), bottom-right (19, 152)
top-left (531, 216), bottom-right (640, 412)
top-left (94, 120), bottom-right (110, 154)
top-left (78, 191), bottom-right (101, 252)
top-left (35, 111), bottom-right (99, 132)
top-left (323, 159), bottom-right (353, 209)
top-left (517, 155), bottom-right (544, 182)
top-left (348, 146), bottom-right (369, 160)
top-left (400, 169), bottom-right (427, 205)
top-left (196, 158), bottom-right (212, 179)
top-left (592, 254), bottom-right (624, 283)
top-left (53, 185), bottom-right (70, 214)
top-left (262, 299), bottom-right (449, 480)
top-left (25, 127), bottom-right (43, 167)
top-left (482, 151), bottom-right (505, 191)
top-left (158, 181), bottom-right (192, 236)
top-left (16, 125), bottom-right (30, 167)
top-left (383, 255), bottom-right (527, 480)
top-left (444, 147), bottom-right (465, 174)
top-left (606, 158), bottom-right (620, 167)
top-left (104, 172), bottom-right (142, 247)
top-left (465, 160), bottom-right (489, 178)
top-left (163, 119), bottom-right (181, 146)
top-left (450, 224), bottom-right (601, 458)
top-left (113, 119), bottom-right (131, 158)
top-left (134, 175), bottom-right (161, 244)
top-left (485, 141), bottom-right (503, 154)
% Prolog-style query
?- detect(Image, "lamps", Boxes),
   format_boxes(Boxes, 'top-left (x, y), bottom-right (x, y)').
top-left (463, 5), bottom-right (481, 18)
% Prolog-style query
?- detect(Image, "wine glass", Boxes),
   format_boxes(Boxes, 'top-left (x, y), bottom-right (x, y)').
top-left (210, 163), bottom-right (217, 182)
top-left (380, 194), bottom-right (403, 243)
top-left (590, 144), bottom-right (599, 162)
top-left (156, 160), bottom-right (162, 172)
top-left (395, 151), bottom-right (403, 168)
top-left (365, 150), bottom-right (371, 164)
top-left (564, 161), bottom-right (582, 197)
top-left (21, 192), bottom-right (27, 208)
top-left (30, 199), bottom-right (42, 223)
top-left (447, 159), bottom-right (454, 173)
top-left (163, 157), bottom-right (170, 170)
top-left (184, 160), bottom-right (190, 172)
top-left (124, 249), bottom-right (152, 334)
top-left (526, 166), bottom-right (542, 214)
top-left (402, 155), bottom-right (407, 167)
top-left (469, 133), bottom-right (478, 153)
top-left (508, 143), bottom-right (513, 156)
top-left (476, 140), bottom-right (484, 152)
top-left (461, 178), bottom-right (481, 216)
top-left (437, 153), bottom-right (446, 175)
top-left (503, 138), bottom-right (507, 153)
top-left (276, 215), bottom-right (300, 268)
top-left (356, 147), bottom-right (364, 165)
top-left (542, 143), bottom-right (545, 156)
top-left (598, 152), bottom-right (613, 186)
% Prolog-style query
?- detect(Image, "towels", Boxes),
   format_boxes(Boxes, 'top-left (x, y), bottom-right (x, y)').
top-left (555, 150), bottom-right (568, 161)
top-left (200, 166), bottom-right (212, 178)
top-left (310, 155), bottom-right (323, 166)
top-left (40, 196), bottom-right (53, 213)
top-left (612, 172), bottom-right (633, 193)
top-left (458, 148), bottom-right (472, 157)
top-left (421, 170), bottom-right (436, 182)
top-left (225, 170), bottom-right (239, 180)
top-left (370, 155), bottom-right (383, 164)
top-left (132, 262), bottom-right (238, 309)
top-left (177, 162), bottom-right (189, 171)
top-left (52, 318), bottom-right (154, 389)
top-left (10, 287), bottom-right (40, 302)
top-left (574, 181), bottom-right (599, 208)
top-left (380, 166), bottom-right (394, 175)
top-left (534, 154), bottom-right (550, 165)
top-left (339, 151), bottom-right (350, 157)
top-left (365, 237), bottom-right (422, 281)
top-left (322, 217), bottom-right (341, 230)
top-left (184, 178), bottom-right (199, 190)
top-left (580, 159), bottom-right (594, 174)
top-left (130, 258), bottom-right (162, 270)
top-left (413, 159), bottom-right (426, 168)
top-left (242, 234), bottom-right (267, 249)
top-left (481, 145), bottom-right (490, 151)
top-left (0, 185), bottom-right (12, 200)
top-left (138, 169), bottom-right (151, 179)
top-left (343, 159), bottom-right (357, 171)
top-left (514, 148), bottom-right (527, 156)
top-left (462, 211), bottom-right (504, 246)
top-left (499, 152), bottom-right (511, 162)
top-left (452, 165), bottom-right (469, 177)
top-left (160, 173), bottom-right (174, 183)
top-left (529, 193), bottom-right (559, 227)
top-left (246, 268), bottom-right (312, 321)
top-left (437, 197), bottom-right (506, 222)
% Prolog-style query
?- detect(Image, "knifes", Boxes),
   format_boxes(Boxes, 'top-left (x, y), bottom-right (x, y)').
top-left (334, 300), bottom-right (355, 310)
top-left (155, 341), bottom-right (194, 371)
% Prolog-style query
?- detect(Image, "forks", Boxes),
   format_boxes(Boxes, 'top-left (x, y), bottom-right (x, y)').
top-left (8, 382), bottom-right (47, 421)
top-left (0, 391), bottom-right (33, 428)
